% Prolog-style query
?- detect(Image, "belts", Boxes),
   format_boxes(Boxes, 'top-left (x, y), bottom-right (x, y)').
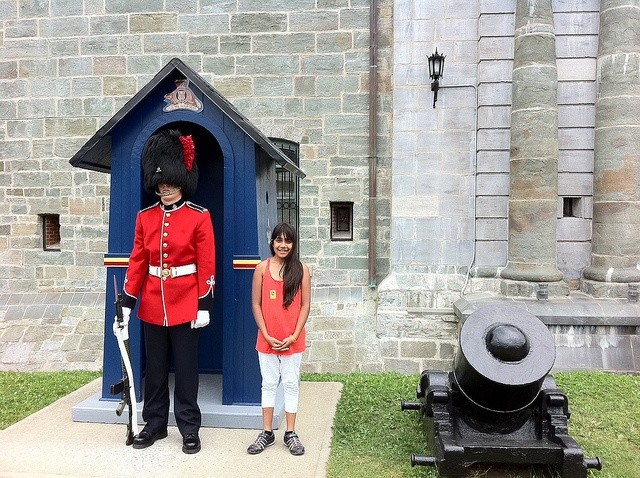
top-left (148, 264), bottom-right (198, 281)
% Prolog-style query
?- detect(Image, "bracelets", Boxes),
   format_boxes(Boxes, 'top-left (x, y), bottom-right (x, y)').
top-left (289, 334), bottom-right (297, 344)
top-left (246, 223), bottom-right (311, 456)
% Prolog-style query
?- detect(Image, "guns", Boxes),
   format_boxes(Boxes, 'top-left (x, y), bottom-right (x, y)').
top-left (110, 274), bottom-right (138, 447)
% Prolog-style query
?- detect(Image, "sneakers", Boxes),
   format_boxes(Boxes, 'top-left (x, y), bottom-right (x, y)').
top-left (247, 431), bottom-right (275, 454)
top-left (283, 431), bottom-right (304, 455)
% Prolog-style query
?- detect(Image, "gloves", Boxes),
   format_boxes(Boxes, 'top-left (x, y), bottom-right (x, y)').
top-left (113, 307), bottom-right (132, 340)
top-left (190, 310), bottom-right (210, 329)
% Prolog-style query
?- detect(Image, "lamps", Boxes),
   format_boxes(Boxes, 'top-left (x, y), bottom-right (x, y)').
top-left (425, 46), bottom-right (447, 108)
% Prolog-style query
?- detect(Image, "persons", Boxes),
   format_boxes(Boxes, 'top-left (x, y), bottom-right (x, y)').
top-left (112, 129), bottom-right (216, 455)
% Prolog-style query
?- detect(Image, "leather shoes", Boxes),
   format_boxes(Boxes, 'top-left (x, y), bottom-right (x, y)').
top-left (133, 428), bottom-right (167, 449)
top-left (182, 433), bottom-right (201, 454)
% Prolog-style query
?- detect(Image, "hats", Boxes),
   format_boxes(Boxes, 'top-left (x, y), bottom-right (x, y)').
top-left (142, 130), bottom-right (197, 199)
